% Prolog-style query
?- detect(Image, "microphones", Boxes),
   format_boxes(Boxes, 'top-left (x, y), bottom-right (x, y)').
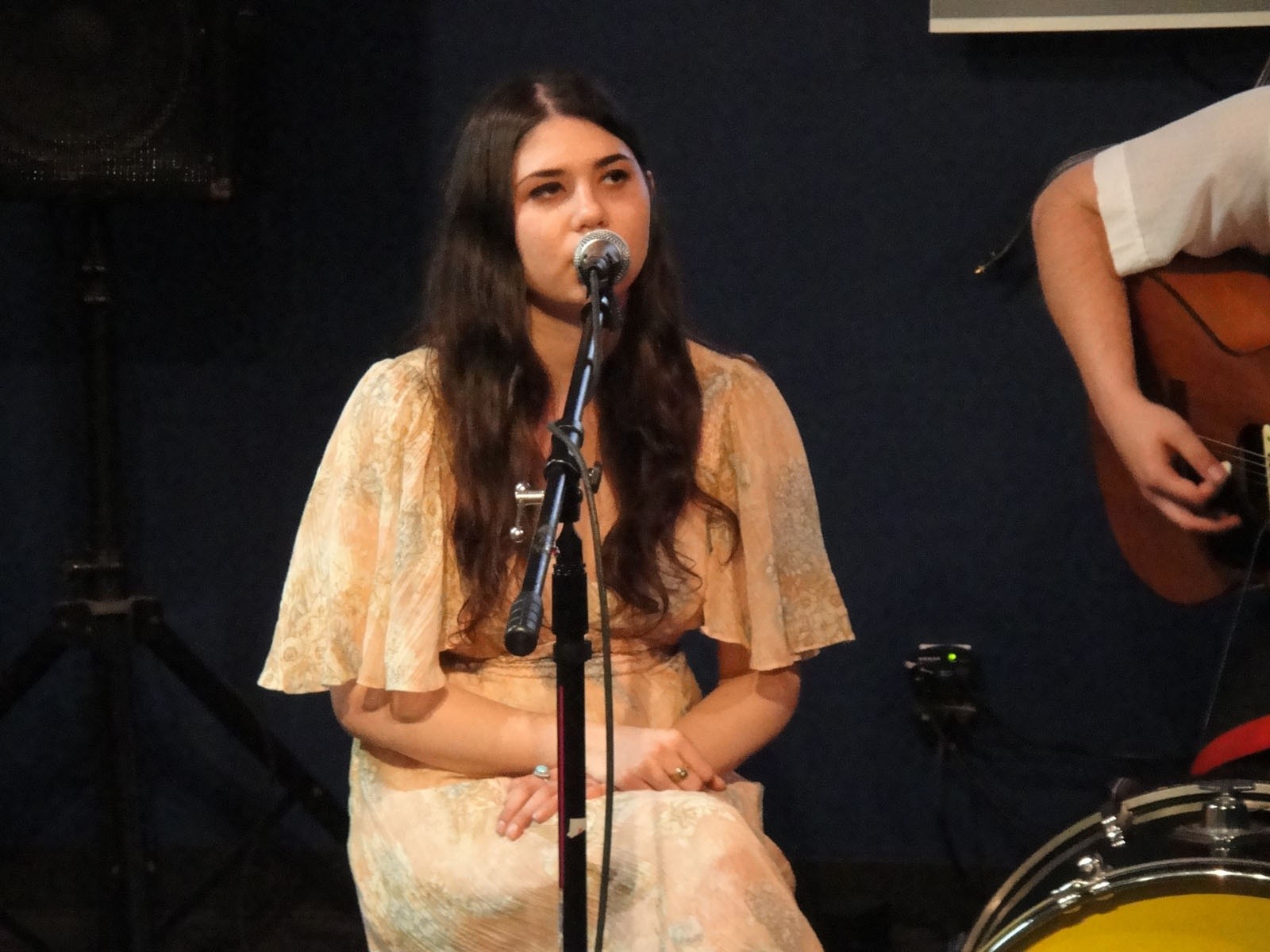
top-left (572, 227), bottom-right (631, 289)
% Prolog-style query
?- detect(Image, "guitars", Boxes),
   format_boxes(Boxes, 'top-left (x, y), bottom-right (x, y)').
top-left (1088, 248), bottom-right (1270, 607)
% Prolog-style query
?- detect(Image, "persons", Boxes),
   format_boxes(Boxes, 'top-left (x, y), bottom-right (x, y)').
top-left (1032, 83), bottom-right (1270, 780)
top-left (259, 68), bottom-right (858, 952)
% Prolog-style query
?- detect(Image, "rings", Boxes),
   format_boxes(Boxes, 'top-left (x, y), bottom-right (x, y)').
top-left (535, 766), bottom-right (552, 783)
top-left (669, 764), bottom-right (691, 783)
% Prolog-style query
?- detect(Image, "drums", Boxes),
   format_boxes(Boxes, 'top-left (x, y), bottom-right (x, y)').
top-left (958, 776), bottom-right (1270, 952)
